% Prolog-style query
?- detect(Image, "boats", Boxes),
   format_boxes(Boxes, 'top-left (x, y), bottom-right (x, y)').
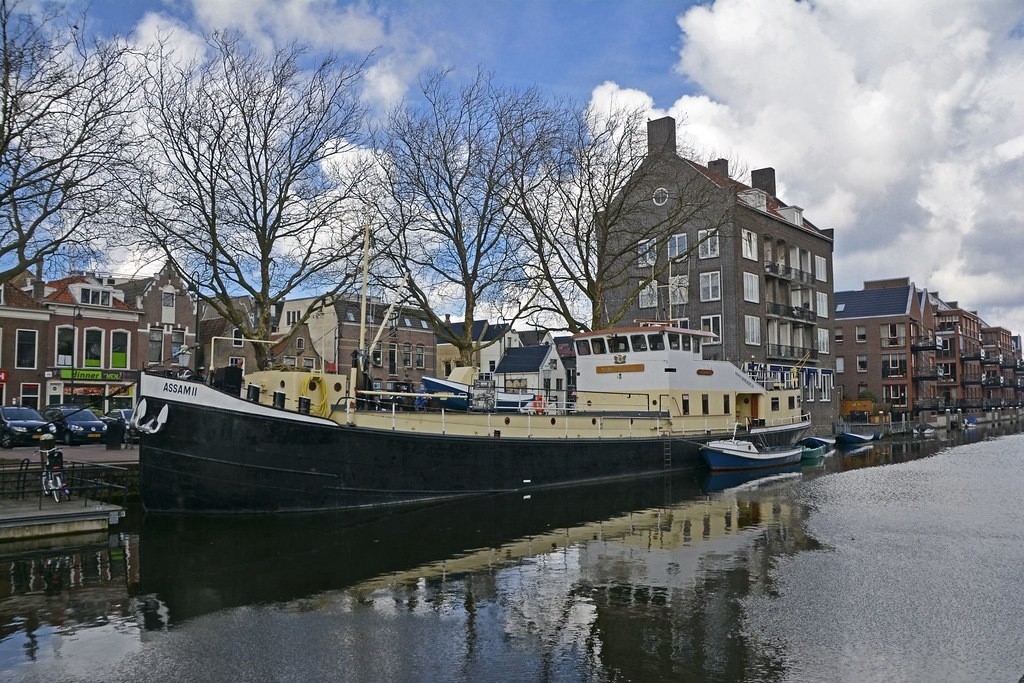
top-left (422, 376), bottom-right (536, 412)
top-left (912, 424), bottom-right (935, 434)
top-left (801, 445), bottom-right (826, 459)
top-left (801, 436), bottom-right (837, 448)
top-left (699, 439), bottom-right (802, 470)
top-left (137, 320), bottom-right (813, 511)
top-left (962, 416), bottom-right (977, 427)
top-left (843, 432), bottom-right (874, 442)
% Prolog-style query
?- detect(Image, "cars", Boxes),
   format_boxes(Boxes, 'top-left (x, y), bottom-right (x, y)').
top-left (100, 408), bottom-right (135, 444)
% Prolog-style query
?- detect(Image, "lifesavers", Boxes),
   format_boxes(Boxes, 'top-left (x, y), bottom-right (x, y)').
top-left (534, 398), bottom-right (546, 412)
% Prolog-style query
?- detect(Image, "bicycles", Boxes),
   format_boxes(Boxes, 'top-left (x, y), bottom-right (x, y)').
top-left (33, 448), bottom-right (70, 503)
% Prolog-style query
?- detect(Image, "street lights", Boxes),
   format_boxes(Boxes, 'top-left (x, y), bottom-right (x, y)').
top-left (188, 271), bottom-right (200, 377)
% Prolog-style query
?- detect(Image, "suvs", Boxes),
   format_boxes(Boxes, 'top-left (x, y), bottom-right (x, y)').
top-left (0, 406), bottom-right (56, 448)
top-left (38, 404), bottom-right (109, 445)
